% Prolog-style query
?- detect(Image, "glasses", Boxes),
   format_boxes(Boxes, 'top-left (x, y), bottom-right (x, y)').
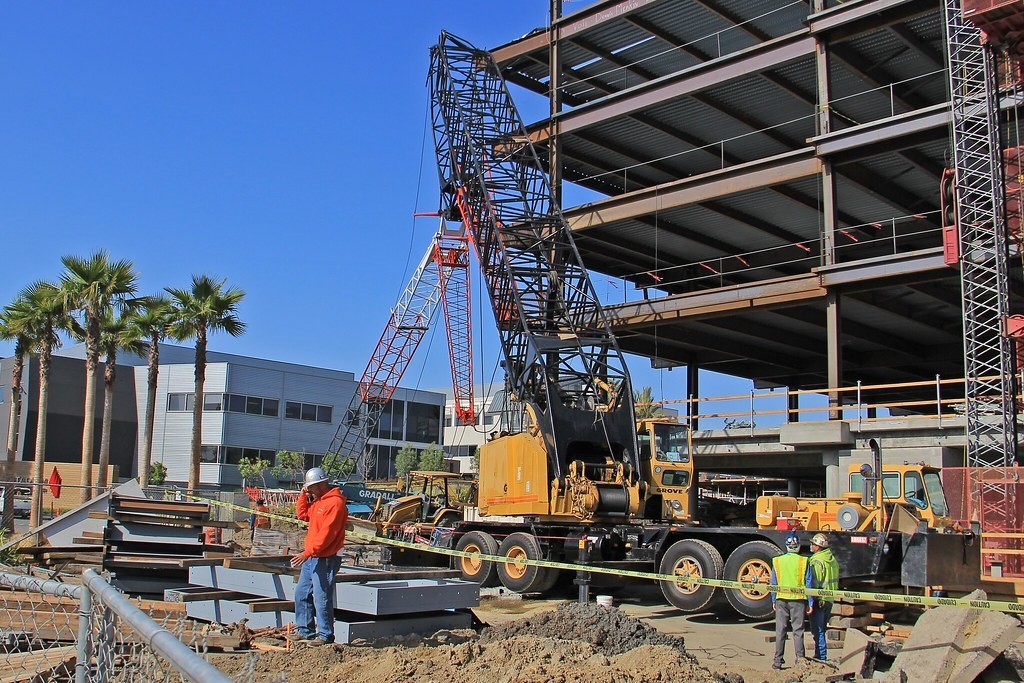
top-left (811, 542), bottom-right (817, 546)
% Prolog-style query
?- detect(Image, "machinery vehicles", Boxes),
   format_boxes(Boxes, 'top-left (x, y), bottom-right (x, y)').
top-left (755, 461), bottom-right (982, 537)
top-left (372, 30), bottom-right (1017, 627)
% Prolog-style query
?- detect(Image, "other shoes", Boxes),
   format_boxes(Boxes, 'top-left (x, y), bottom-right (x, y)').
top-left (307, 637), bottom-right (335, 647)
top-left (285, 631), bottom-right (315, 641)
top-left (772, 663), bottom-right (781, 671)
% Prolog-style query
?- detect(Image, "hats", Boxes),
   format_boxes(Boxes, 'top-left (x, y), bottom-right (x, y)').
top-left (785, 534), bottom-right (800, 552)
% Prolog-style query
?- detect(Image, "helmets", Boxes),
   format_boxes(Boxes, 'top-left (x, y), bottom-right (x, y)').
top-left (809, 533), bottom-right (828, 548)
top-left (302, 468), bottom-right (329, 488)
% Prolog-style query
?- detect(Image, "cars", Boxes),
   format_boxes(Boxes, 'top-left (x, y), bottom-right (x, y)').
top-left (0, 486), bottom-right (32, 518)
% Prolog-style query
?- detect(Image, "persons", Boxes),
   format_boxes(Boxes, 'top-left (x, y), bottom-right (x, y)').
top-left (250, 498), bottom-right (271, 543)
top-left (772, 533), bottom-right (839, 669)
top-left (286, 468), bottom-right (348, 647)
